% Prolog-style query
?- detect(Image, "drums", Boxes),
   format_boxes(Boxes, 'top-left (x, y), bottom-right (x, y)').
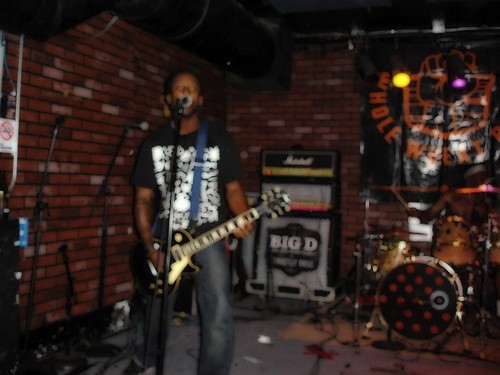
top-left (372, 254), bottom-right (464, 344)
top-left (367, 239), bottom-right (420, 278)
top-left (431, 216), bottom-right (480, 268)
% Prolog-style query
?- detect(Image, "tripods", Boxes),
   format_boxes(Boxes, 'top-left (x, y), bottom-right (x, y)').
top-left (434, 193), bottom-right (500, 356)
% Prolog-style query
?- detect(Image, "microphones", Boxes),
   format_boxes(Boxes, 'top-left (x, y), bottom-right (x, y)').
top-left (122, 121), bottom-right (149, 131)
top-left (180, 96), bottom-right (192, 108)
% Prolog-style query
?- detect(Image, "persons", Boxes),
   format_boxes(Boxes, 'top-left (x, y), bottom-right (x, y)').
top-left (409, 159), bottom-right (500, 325)
top-left (128, 64), bottom-right (254, 375)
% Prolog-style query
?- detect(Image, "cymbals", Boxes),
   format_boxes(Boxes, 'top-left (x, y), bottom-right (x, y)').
top-left (456, 184), bottom-right (500, 194)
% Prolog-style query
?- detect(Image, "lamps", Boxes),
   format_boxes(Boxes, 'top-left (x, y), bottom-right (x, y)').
top-left (355, 53), bottom-right (382, 88)
top-left (390, 54), bottom-right (410, 88)
top-left (445, 54), bottom-right (469, 90)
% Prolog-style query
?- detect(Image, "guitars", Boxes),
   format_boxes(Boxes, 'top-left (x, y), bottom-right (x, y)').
top-left (128, 186), bottom-right (292, 298)
